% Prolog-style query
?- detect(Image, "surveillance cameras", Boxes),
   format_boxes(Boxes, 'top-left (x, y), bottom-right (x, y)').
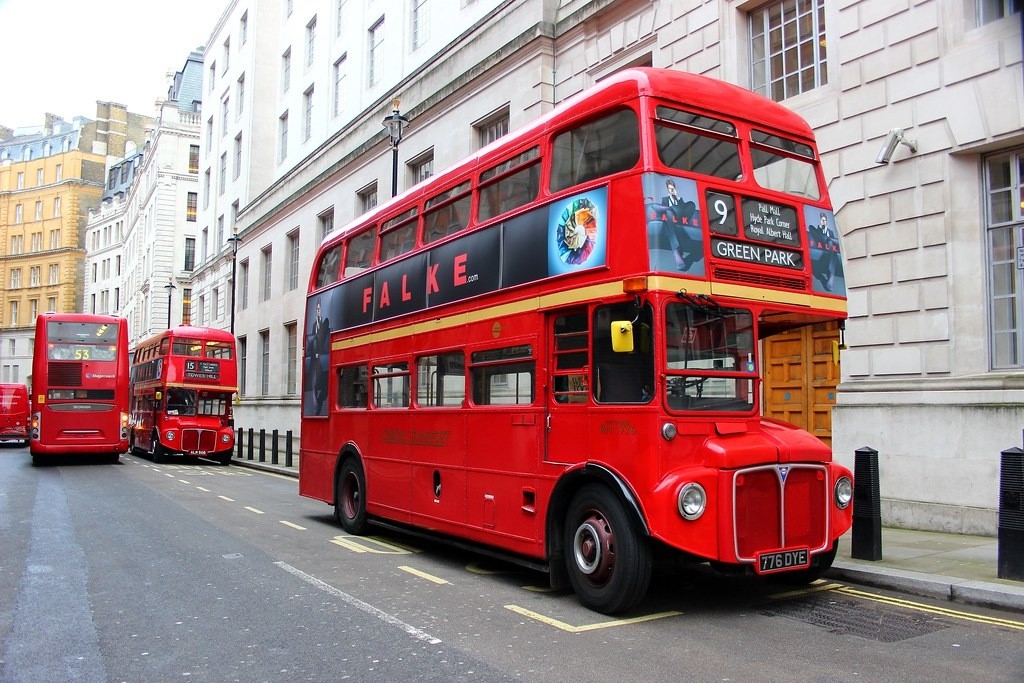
top-left (876, 127), bottom-right (904, 163)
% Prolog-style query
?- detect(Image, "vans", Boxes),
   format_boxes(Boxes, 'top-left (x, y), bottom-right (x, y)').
top-left (0, 384), bottom-right (30, 444)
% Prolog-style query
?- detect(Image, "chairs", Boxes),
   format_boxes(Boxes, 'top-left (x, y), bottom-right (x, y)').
top-left (400, 191), bottom-right (530, 256)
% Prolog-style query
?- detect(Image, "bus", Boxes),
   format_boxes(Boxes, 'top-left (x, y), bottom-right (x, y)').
top-left (129, 324), bottom-right (240, 466)
top-left (31, 313), bottom-right (130, 465)
top-left (300, 66), bottom-right (855, 617)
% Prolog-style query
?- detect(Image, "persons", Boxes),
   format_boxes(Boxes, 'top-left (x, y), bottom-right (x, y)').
top-left (167, 387), bottom-right (188, 414)
top-left (651, 179), bottom-right (696, 271)
top-left (809, 212), bottom-right (840, 290)
top-left (307, 296), bottom-right (330, 415)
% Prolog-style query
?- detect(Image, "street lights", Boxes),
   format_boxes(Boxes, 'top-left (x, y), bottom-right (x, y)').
top-left (226, 227), bottom-right (242, 428)
top-left (164, 277), bottom-right (177, 330)
top-left (382, 100), bottom-right (411, 198)
